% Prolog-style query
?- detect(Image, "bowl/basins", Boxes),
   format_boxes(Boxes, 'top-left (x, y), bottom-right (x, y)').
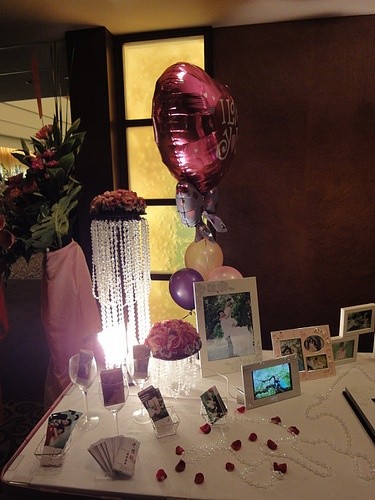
top-left (152, 405), bottom-right (180, 439)
top-left (34, 433), bottom-right (70, 467)
top-left (200, 397), bottom-right (228, 425)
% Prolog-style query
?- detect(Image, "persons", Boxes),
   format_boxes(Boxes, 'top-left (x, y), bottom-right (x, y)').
top-left (309, 312), bottom-right (372, 370)
top-left (47, 418), bottom-right (72, 445)
top-left (218, 294), bottom-right (240, 359)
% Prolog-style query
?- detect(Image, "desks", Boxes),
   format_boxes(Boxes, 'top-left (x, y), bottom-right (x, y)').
top-left (0, 350), bottom-right (375, 500)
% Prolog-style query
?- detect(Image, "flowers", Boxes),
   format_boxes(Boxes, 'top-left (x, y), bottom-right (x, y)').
top-left (145, 321), bottom-right (202, 360)
top-left (0, 115), bottom-right (85, 280)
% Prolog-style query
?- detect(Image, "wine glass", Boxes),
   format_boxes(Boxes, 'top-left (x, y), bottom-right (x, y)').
top-left (125, 352), bottom-right (153, 424)
top-left (67, 353), bottom-right (100, 431)
top-left (99, 375), bottom-right (130, 436)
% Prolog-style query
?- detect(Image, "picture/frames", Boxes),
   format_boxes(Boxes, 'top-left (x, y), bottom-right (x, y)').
top-left (339, 303), bottom-right (375, 338)
top-left (271, 325), bottom-right (336, 382)
top-left (331, 335), bottom-right (359, 366)
top-left (193, 277), bottom-right (263, 378)
top-left (241, 353), bottom-right (301, 409)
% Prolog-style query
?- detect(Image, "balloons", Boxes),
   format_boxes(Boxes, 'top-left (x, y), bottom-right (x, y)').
top-left (205, 265), bottom-right (244, 281)
top-left (185, 238), bottom-right (223, 281)
top-left (152, 62), bottom-right (237, 195)
top-left (175, 179), bottom-right (218, 229)
top-left (170, 267), bottom-right (203, 311)
